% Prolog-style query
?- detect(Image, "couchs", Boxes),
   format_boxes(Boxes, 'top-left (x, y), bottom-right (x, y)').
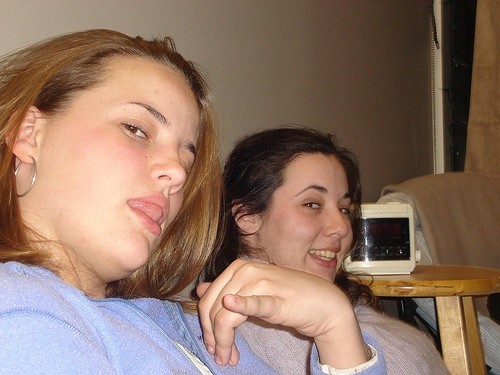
top-left (377, 172), bottom-right (500, 374)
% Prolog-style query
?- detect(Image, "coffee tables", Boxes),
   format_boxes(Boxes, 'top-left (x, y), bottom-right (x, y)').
top-left (348, 264), bottom-right (500, 375)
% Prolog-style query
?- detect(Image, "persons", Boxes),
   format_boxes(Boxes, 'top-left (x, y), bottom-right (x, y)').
top-left (0, 27), bottom-right (388, 375)
top-left (189, 126), bottom-right (449, 375)
